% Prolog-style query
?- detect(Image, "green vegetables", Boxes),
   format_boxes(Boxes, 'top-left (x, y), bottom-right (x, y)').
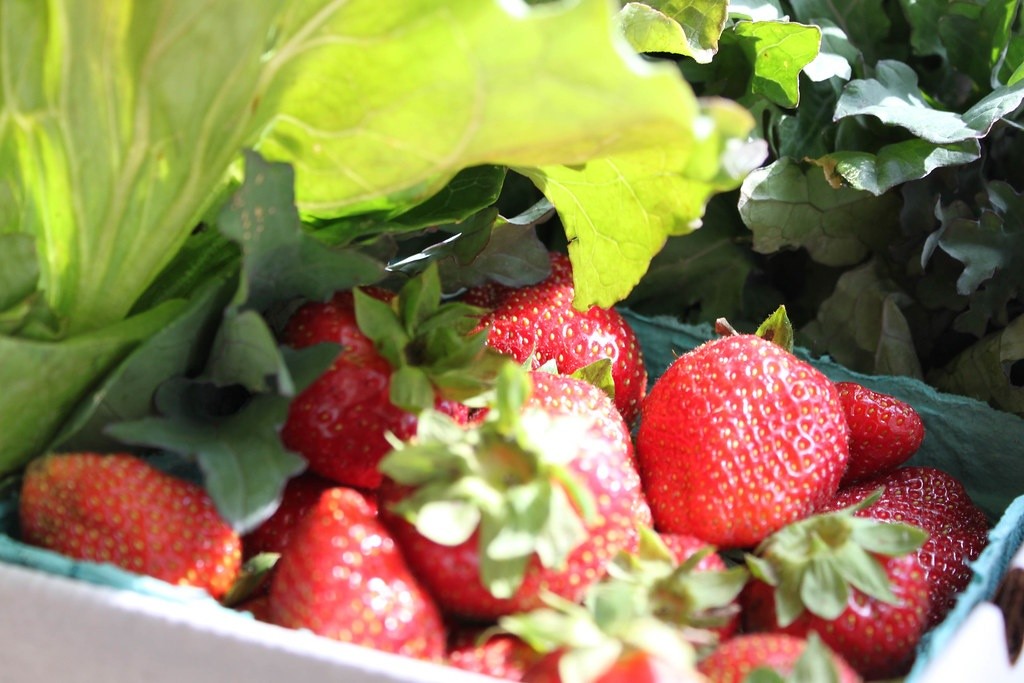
top-left (50, 0), bottom-right (1024, 536)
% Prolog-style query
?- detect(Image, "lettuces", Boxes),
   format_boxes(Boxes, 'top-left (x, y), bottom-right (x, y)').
top-left (0, 0), bottom-right (731, 473)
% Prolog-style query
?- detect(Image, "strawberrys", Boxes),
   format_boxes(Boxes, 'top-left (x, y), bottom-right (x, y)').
top-left (18, 247), bottom-right (1004, 683)
